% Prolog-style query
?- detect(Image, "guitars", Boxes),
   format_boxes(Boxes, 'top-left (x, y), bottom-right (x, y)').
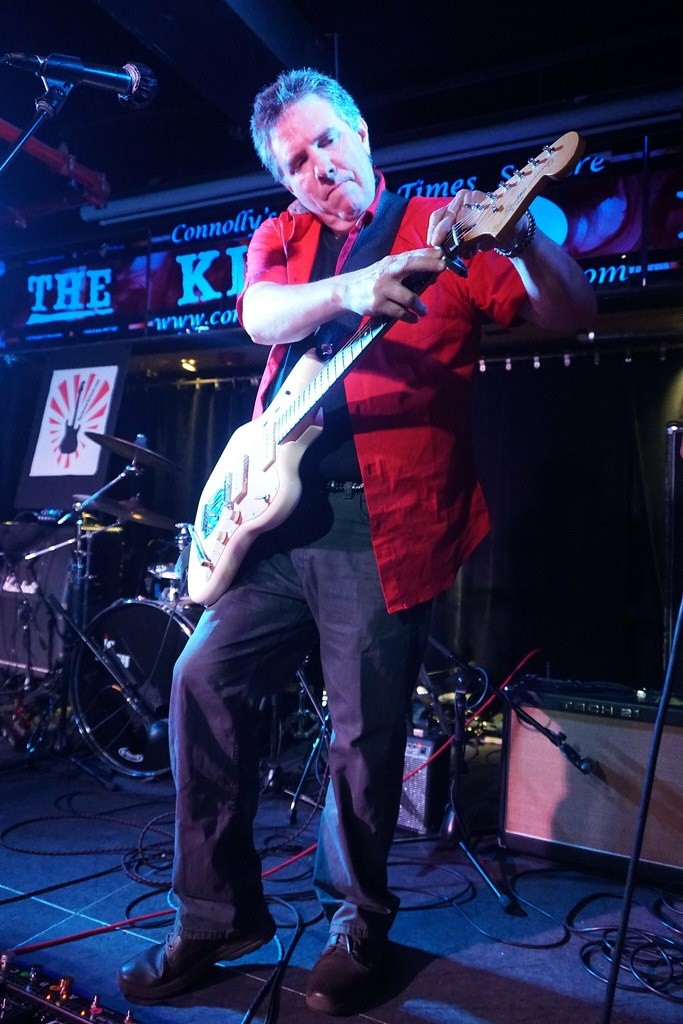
top-left (183, 129), bottom-right (584, 597)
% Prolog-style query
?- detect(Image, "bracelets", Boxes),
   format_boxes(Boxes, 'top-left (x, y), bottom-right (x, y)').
top-left (493, 209), bottom-right (536, 258)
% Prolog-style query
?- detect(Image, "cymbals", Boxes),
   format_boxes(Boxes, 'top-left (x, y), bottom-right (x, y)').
top-left (84, 427), bottom-right (173, 466)
top-left (71, 493), bottom-right (183, 529)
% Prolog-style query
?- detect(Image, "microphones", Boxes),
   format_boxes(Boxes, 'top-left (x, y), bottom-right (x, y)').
top-left (559, 743), bottom-right (597, 774)
top-left (2, 53), bottom-right (159, 109)
top-left (122, 691), bottom-right (169, 742)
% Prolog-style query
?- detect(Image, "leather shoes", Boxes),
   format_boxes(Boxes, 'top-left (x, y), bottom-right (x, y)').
top-left (118, 897), bottom-right (277, 1001)
top-left (307, 933), bottom-right (388, 1012)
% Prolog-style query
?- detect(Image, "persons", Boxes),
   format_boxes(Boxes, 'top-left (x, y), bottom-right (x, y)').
top-left (118, 66), bottom-right (598, 1014)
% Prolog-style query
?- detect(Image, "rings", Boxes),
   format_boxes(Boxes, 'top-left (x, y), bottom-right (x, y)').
top-left (460, 204), bottom-right (472, 209)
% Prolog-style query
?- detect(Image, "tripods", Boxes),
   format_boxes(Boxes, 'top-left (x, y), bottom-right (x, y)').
top-left (279, 654), bottom-right (325, 742)
top-left (0, 457), bottom-right (139, 794)
top-left (394, 633), bottom-right (571, 912)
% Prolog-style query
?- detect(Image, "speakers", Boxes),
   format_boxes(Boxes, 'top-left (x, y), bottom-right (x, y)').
top-left (395, 734), bottom-right (448, 836)
top-left (498, 688), bottom-right (683, 883)
top-left (0, 521), bottom-right (129, 681)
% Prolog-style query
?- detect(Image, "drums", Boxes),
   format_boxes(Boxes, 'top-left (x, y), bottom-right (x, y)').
top-left (146, 560), bottom-right (202, 603)
top-left (69, 594), bottom-right (209, 781)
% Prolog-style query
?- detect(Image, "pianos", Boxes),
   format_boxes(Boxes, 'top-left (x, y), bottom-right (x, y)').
top-left (0, 507), bottom-right (76, 524)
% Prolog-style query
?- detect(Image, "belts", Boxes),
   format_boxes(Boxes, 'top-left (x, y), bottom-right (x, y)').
top-left (315, 479), bottom-right (364, 493)
